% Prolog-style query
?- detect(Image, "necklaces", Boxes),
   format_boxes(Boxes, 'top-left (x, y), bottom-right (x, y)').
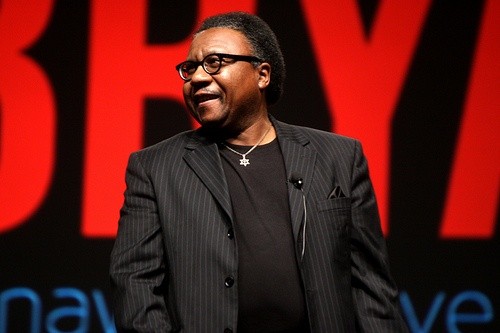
top-left (219, 121), bottom-right (272, 166)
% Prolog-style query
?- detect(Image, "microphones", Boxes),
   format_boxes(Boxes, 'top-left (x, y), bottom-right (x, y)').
top-left (291, 173), bottom-right (303, 190)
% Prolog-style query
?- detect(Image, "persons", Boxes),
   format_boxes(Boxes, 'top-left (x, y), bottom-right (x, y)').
top-left (106, 11), bottom-right (410, 333)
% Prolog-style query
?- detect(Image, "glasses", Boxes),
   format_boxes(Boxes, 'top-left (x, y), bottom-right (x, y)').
top-left (176, 53), bottom-right (264, 81)
